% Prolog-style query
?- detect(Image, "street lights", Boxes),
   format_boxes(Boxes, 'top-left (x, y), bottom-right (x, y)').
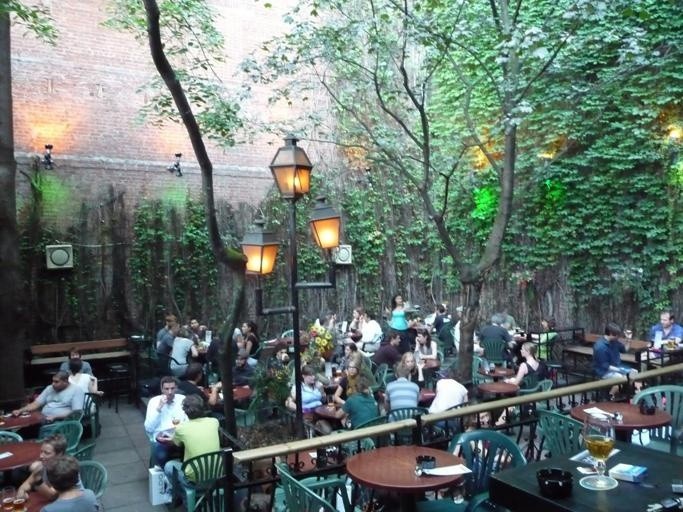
top-left (242, 133), bottom-right (341, 436)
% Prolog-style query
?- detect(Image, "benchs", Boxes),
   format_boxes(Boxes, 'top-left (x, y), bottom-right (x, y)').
top-left (558, 367), bottom-right (599, 406)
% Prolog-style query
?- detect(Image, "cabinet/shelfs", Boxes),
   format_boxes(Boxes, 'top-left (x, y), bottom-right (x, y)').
top-left (530, 328), bottom-right (584, 362)
top-left (647, 344), bottom-right (682, 385)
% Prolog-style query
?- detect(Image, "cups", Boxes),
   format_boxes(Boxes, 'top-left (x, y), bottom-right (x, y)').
top-left (0, 486), bottom-right (13, 512)
top-left (20, 400), bottom-right (30, 417)
top-left (667, 340), bottom-right (674, 350)
top-left (490, 363), bottom-right (495, 375)
top-left (208, 383), bottom-right (215, 393)
top-left (14, 488), bottom-right (28, 512)
top-left (519, 331), bottom-right (525, 337)
top-left (414, 351), bottom-right (419, 364)
top-left (326, 394), bottom-right (334, 410)
top-left (515, 328), bottom-right (520, 333)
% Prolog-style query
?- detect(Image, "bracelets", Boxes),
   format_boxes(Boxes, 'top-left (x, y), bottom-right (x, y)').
top-left (34, 477), bottom-right (43, 487)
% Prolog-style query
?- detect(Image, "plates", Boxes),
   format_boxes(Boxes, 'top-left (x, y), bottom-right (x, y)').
top-left (405, 310), bottom-right (419, 312)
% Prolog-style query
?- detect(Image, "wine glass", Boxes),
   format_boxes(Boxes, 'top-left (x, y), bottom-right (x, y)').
top-left (172, 413), bottom-right (181, 429)
top-left (579, 414), bottom-right (617, 492)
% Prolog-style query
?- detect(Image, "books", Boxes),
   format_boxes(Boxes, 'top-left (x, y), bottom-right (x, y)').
top-left (607, 464), bottom-right (648, 483)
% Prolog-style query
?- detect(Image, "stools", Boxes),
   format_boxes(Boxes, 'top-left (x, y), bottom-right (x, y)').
top-left (108, 365), bottom-right (139, 412)
top-left (546, 361), bottom-right (570, 408)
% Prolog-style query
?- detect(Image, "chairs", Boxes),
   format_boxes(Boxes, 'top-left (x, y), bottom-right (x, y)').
top-left (156, 322), bottom-right (555, 512)
top-left (538, 410), bottom-right (606, 459)
top-left (0, 386), bottom-right (107, 512)
top-left (632, 384), bottom-right (683, 455)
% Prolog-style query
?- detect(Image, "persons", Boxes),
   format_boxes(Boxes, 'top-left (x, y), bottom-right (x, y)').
top-left (39, 455), bottom-right (100, 512)
top-left (151, 315), bottom-right (296, 379)
top-left (12, 371), bottom-right (85, 439)
top-left (589, 322), bottom-right (643, 400)
top-left (639, 312), bottom-right (682, 372)
top-left (144, 350), bottom-right (256, 470)
top-left (16, 433), bottom-right (84, 500)
top-left (59, 346), bottom-right (92, 376)
top-left (285, 294), bottom-right (559, 464)
top-left (67, 358), bottom-right (104, 416)
top-left (163, 393), bottom-right (226, 512)
